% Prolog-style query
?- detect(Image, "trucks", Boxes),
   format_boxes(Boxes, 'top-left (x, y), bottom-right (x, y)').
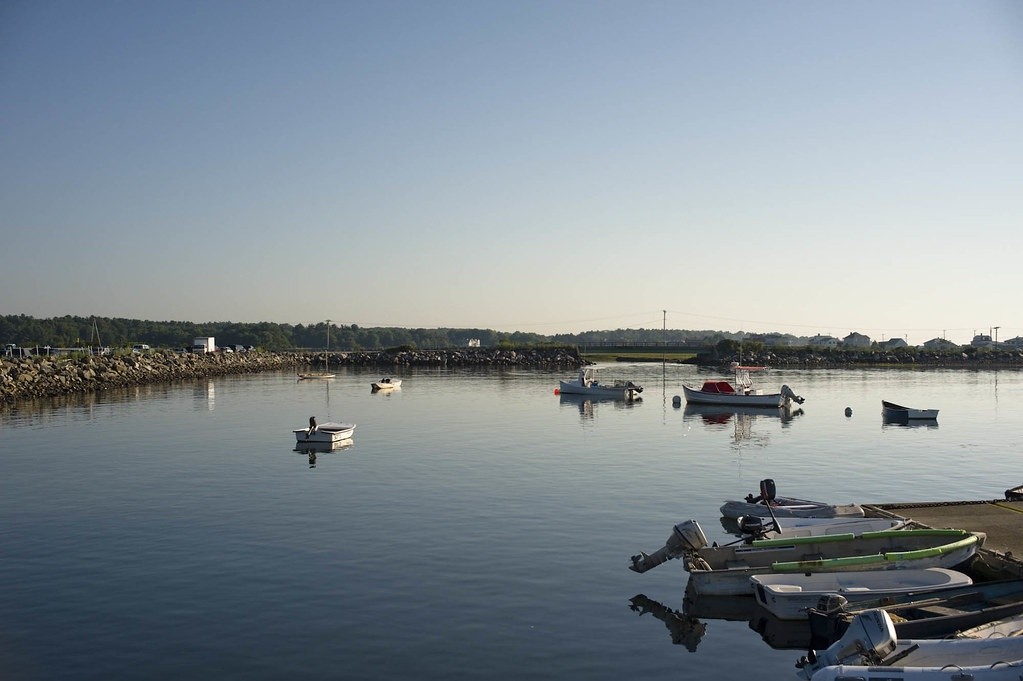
top-left (193, 337), bottom-right (215, 355)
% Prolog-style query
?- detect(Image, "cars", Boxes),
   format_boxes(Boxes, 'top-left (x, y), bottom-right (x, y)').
top-left (246, 344), bottom-right (257, 352)
top-left (134, 344), bottom-right (149, 352)
top-left (233, 344), bottom-right (246, 352)
top-left (224, 346), bottom-right (233, 355)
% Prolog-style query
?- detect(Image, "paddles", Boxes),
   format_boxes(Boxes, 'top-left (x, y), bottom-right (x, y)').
top-left (836, 589), bottom-right (984, 618)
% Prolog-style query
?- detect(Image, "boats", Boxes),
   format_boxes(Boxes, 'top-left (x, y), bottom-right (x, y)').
top-left (297, 370), bottom-right (337, 381)
top-left (794, 609), bottom-right (1023, 681)
top-left (293, 415), bottom-right (357, 452)
top-left (682, 362), bottom-right (806, 408)
top-left (371, 378), bottom-right (404, 393)
top-left (797, 579), bottom-right (1022, 644)
top-left (558, 367), bottom-right (643, 401)
top-left (720, 477), bottom-right (868, 521)
top-left (748, 569), bottom-right (977, 620)
top-left (629, 520), bottom-right (987, 595)
top-left (881, 399), bottom-right (940, 420)
